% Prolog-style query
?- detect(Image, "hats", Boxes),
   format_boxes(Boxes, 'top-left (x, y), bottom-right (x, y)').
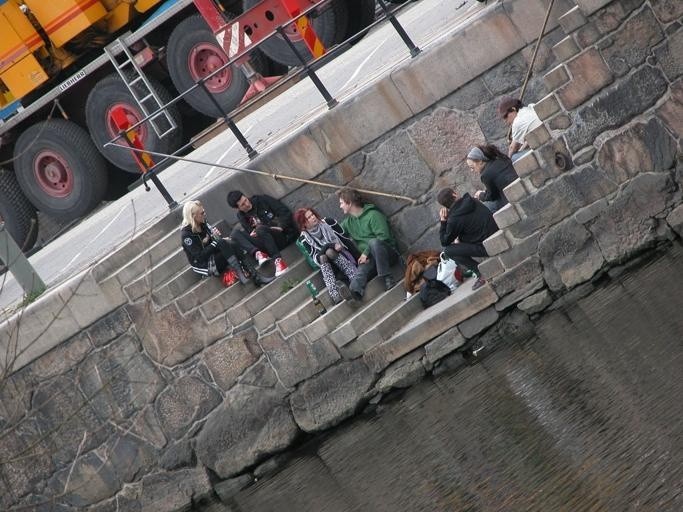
top-left (499, 97), bottom-right (521, 118)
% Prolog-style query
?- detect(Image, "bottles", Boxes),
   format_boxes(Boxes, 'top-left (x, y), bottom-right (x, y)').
top-left (311, 294), bottom-right (327, 314)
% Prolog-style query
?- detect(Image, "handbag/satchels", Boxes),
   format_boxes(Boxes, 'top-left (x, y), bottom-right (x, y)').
top-left (420, 280), bottom-right (451, 309)
top-left (437, 252), bottom-right (463, 292)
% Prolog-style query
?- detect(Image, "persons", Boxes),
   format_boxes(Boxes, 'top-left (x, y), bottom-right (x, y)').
top-left (179, 198), bottom-right (276, 289)
top-left (291, 205), bottom-right (357, 304)
top-left (225, 189), bottom-right (300, 277)
top-left (436, 187), bottom-right (500, 290)
top-left (335, 188), bottom-right (400, 305)
top-left (465, 140), bottom-right (519, 213)
top-left (498, 94), bottom-right (543, 163)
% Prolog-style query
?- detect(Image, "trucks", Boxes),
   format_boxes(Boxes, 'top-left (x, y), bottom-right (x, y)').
top-left (0, 0), bottom-right (382, 279)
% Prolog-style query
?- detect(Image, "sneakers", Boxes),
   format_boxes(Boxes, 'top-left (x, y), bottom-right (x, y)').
top-left (385, 275), bottom-right (396, 291)
top-left (255, 251), bottom-right (269, 266)
top-left (472, 275), bottom-right (486, 290)
top-left (351, 291), bottom-right (362, 307)
top-left (275, 257), bottom-right (288, 277)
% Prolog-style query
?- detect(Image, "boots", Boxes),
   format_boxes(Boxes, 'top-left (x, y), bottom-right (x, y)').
top-left (243, 256), bottom-right (275, 287)
top-left (320, 263), bottom-right (343, 304)
top-left (333, 254), bottom-right (357, 281)
top-left (227, 255), bottom-right (250, 284)
